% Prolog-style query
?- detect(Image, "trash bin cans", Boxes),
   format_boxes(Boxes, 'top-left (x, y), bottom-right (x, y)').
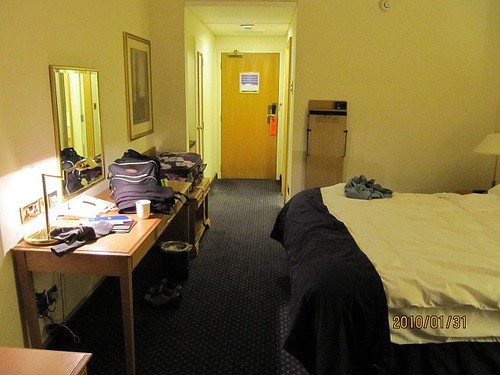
top-left (160, 241), bottom-right (193, 286)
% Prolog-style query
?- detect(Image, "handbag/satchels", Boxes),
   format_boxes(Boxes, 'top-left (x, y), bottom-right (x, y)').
top-left (107, 149), bottom-right (164, 198)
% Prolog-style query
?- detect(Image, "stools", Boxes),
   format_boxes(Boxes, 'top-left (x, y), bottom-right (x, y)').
top-left (0, 345), bottom-right (92, 375)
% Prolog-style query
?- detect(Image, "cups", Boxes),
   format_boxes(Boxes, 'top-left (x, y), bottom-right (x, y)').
top-left (135, 200), bottom-right (151, 219)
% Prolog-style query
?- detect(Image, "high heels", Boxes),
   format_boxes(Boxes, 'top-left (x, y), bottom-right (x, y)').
top-left (142, 284), bottom-right (182, 309)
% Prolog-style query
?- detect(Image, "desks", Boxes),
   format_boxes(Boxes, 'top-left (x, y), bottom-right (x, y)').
top-left (9, 179), bottom-right (192, 375)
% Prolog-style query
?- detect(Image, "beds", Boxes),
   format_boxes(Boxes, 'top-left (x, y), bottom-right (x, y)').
top-left (269, 180), bottom-right (500, 375)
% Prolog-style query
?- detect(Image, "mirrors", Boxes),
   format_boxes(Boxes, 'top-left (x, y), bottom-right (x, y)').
top-left (48, 64), bottom-right (107, 202)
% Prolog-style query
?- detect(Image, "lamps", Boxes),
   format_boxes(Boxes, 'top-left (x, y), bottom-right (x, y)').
top-left (475, 133), bottom-right (500, 189)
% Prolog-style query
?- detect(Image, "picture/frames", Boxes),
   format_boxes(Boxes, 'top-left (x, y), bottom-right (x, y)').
top-left (123, 32), bottom-right (154, 141)
